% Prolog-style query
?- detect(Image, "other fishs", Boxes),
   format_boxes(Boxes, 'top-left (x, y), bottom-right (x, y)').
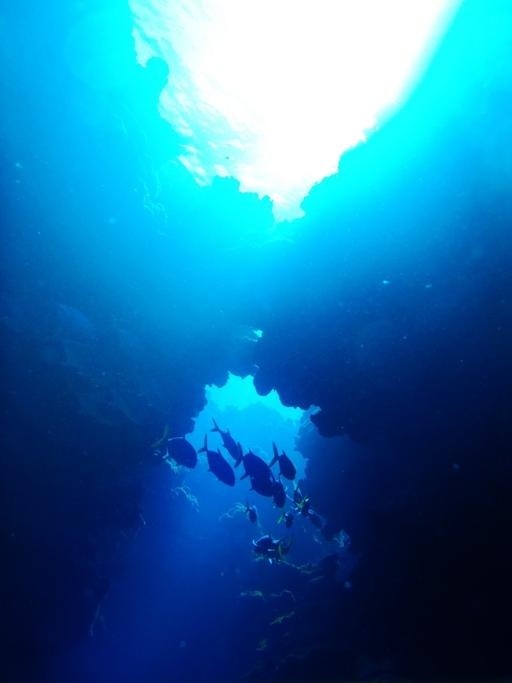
top-left (238, 587), bottom-right (297, 653)
top-left (150, 417), bottom-right (314, 567)
